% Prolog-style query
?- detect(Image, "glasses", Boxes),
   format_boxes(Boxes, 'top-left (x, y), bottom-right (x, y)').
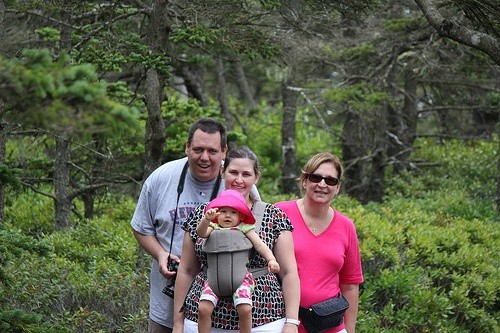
top-left (305, 173), bottom-right (340, 186)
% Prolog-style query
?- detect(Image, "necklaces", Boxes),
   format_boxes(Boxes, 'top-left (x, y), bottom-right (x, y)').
top-left (302, 198), bottom-right (330, 233)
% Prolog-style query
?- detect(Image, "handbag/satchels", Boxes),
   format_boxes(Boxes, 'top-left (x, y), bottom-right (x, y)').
top-left (303, 295), bottom-right (349, 332)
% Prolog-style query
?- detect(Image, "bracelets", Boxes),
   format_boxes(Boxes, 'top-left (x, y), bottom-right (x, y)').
top-left (284, 318), bottom-right (300, 326)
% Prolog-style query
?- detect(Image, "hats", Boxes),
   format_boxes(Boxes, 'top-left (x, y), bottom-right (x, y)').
top-left (204, 190), bottom-right (256, 224)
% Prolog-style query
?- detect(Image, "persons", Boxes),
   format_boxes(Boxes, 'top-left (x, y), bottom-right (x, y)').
top-left (129, 117), bottom-right (262, 333)
top-left (172, 146), bottom-right (301, 333)
top-left (196, 188), bottom-right (280, 333)
top-left (272, 152), bottom-right (364, 333)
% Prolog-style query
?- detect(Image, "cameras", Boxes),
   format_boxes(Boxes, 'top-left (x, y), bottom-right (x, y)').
top-left (162, 259), bottom-right (179, 299)
